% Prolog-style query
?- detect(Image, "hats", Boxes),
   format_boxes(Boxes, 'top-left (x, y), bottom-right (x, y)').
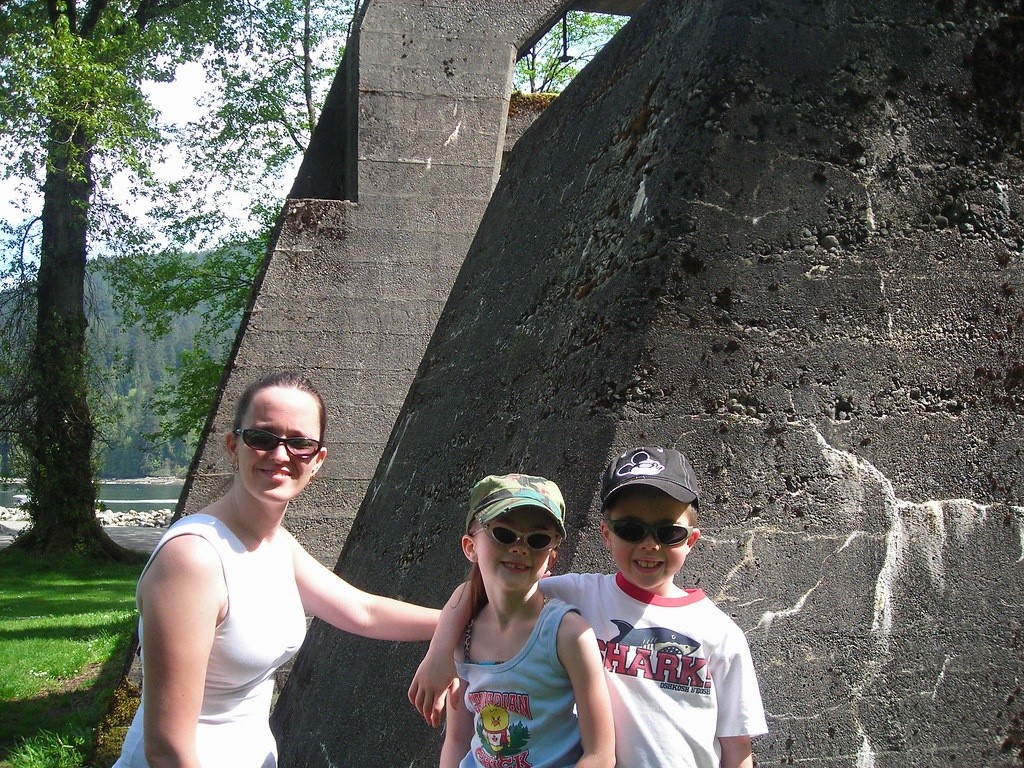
top-left (600, 447), bottom-right (700, 514)
top-left (466, 474), bottom-right (567, 544)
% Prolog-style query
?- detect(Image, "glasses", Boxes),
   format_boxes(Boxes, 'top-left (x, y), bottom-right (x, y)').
top-left (603, 519), bottom-right (694, 546)
top-left (470, 522), bottom-right (562, 550)
top-left (234, 428), bottom-right (321, 457)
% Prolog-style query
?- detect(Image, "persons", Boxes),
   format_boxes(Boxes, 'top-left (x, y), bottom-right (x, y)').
top-left (438, 472), bottom-right (616, 768)
top-left (408, 446), bottom-right (769, 768)
top-left (111, 373), bottom-right (552, 768)
